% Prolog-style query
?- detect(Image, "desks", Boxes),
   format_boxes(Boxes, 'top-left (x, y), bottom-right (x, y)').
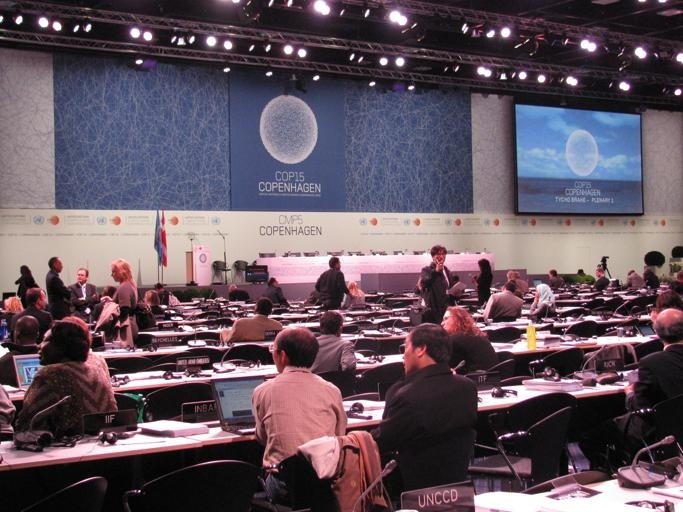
top-left (90, 310), bottom-right (653, 362)
top-left (1, 372), bottom-right (635, 473)
top-left (0, 334), bottom-right (657, 401)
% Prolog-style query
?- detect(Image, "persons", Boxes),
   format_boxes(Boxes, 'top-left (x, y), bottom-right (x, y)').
top-left (13, 322), bottom-right (118, 443)
top-left (154, 283), bottom-right (183, 305)
top-left (306, 287), bottom-right (321, 305)
top-left (314, 257), bottom-right (354, 309)
top-left (418, 245), bottom-right (465, 325)
top-left (620, 269), bottom-right (646, 288)
top-left (0, 316), bottom-right (43, 384)
top-left (14, 265), bottom-right (37, 306)
top-left (668, 271), bottom-right (683, 292)
top-left (484, 283), bottom-right (524, 325)
top-left (341, 281), bottom-right (367, 309)
top-left (547, 267), bottom-right (567, 290)
top-left (251, 327), bottom-right (349, 507)
top-left (308, 311), bottom-right (359, 382)
top-left (220, 297), bottom-right (283, 346)
top-left (67, 267), bottom-right (99, 323)
top-left (506, 269), bottom-right (529, 295)
top-left (99, 259), bottom-right (142, 346)
top-left (371, 324), bottom-right (479, 502)
top-left (472, 259), bottom-right (493, 307)
top-left (572, 269), bottom-right (595, 288)
top-left (528, 284), bottom-right (558, 320)
top-left (228, 284), bottom-right (249, 302)
top-left (8, 287), bottom-right (52, 341)
top-left (59, 315), bottom-right (113, 376)
top-left (46, 258), bottom-right (71, 320)
top-left (594, 263), bottom-right (605, 276)
top-left (91, 285), bottom-right (117, 320)
top-left (264, 277), bottom-right (293, 308)
top-left (642, 268), bottom-right (660, 288)
top-left (507, 280), bottom-right (525, 300)
top-left (650, 287), bottom-right (682, 323)
top-left (441, 306), bottom-right (501, 376)
top-left (579, 308), bottom-right (683, 470)
top-left (142, 290), bottom-right (161, 305)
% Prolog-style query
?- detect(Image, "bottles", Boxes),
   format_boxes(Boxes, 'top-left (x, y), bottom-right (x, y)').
top-left (527, 321), bottom-right (536, 349)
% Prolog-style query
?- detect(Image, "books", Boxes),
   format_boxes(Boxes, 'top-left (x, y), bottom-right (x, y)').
top-left (137, 419), bottom-right (208, 436)
top-left (520, 378), bottom-right (584, 392)
top-left (520, 334), bottom-right (562, 348)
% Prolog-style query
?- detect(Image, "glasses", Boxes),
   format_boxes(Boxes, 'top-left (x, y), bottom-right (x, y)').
top-left (268, 344), bottom-right (285, 353)
top-left (399, 343), bottom-right (419, 353)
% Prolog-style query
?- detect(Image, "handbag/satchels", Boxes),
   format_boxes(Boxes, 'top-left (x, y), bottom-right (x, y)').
top-left (132, 287), bottom-right (157, 329)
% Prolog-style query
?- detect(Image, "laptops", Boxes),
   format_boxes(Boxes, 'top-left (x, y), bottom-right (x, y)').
top-left (532, 279), bottom-right (543, 287)
top-left (12, 354), bottom-right (44, 393)
top-left (634, 324), bottom-right (657, 336)
top-left (209, 375), bottom-right (266, 435)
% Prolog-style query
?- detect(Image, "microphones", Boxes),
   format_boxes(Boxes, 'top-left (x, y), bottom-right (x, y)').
top-left (572, 343), bottom-right (608, 380)
top-left (205, 288), bottom-right (217, 303)
top-left (353, 459), bottom-right (398, 512)
top-left (170, 304), bottom-right (187, 321)
top-left (212, 342), bottom-right (237, 373)
top-left (187, 318), bottom-right (206, 348)
top-left (452, 360), bottom-right (467, 372)
top-left (560, 313), bottom-right (585, 342)
top-left (612, 299), bottom-right (631, 318)
top-left (541, 296), bottom-right (556, 323)
top-left (617, 435), bottom-right (675, 489)
top-left (387, 314), bottom-right (402, 333)
top-left (14, 394), bottom-right (73, 448)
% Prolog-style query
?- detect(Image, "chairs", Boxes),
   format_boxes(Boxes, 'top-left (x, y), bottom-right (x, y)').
top-left (35, 476), bottom-right (111, 512)
top-left (315, 370), bottom-right (355, 398)
top-left (112, 394), bottom-right (139, 424)
top-left (506, 393), bottom-right (577, 432)
top-left (358, 361), bottom-right (404, 394)
top-left (121, 458), bottom-right (273, 512)
top-left (468, 406), bottom-right (572, 494)
top-left (584, 344), bottom-right (635, 371)
top-left (565, 320), bottom-right (601, 336)
top-left (533, 348), bottom-right (584, 375)
top-left (223, 345), bottom-right (273, 365)
top-left (486, 352), bottom-right (517, 377)
top-left (142, 382), bottom-right (213, 427)
top-left (188, 347), bottom-right (224, 368)
top-left (134, 335), bottom-right (159, 347)
top-left (634, 339), bottom-right (664, 358)
top-left (610, 391), bottom-right (656, 468)
top-left (490, 327), bottom-right (520, 342)
top-left (653, 394), bottom-right (683, 431)
top-left (108, 356), bottom-right (153, 377)
top-left (260, 434), bottom-right (408, 512)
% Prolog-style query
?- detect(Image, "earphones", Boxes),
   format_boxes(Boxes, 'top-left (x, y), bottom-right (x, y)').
top-left (625, 326), bottom-right (639, 337)
top-left (491, 385), bottom-right (518, 398)
top-left (111, 374), bottom-right (131, 384)
top-left (162, 368), bottom-right (182, 379)
top-left (97, 430), bottom-right (133, 444)
top-left (368, 354), bottom-right (386, 363)
top-left (238, 359), bottom-right (263, 369)
top-left (185, 365), bottom-right (201, 376)
top-left (142, 343), bottom-right (159, 353)
top-left (625, 500), bottom-right (675, 512)
top-left (23, 430), bottom-right (53, 448)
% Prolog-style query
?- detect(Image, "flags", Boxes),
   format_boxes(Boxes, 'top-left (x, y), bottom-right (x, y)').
top-left (153, 209), bottom-right (162, 267)
top-left (161, 208), bottom-right (168, 267)
